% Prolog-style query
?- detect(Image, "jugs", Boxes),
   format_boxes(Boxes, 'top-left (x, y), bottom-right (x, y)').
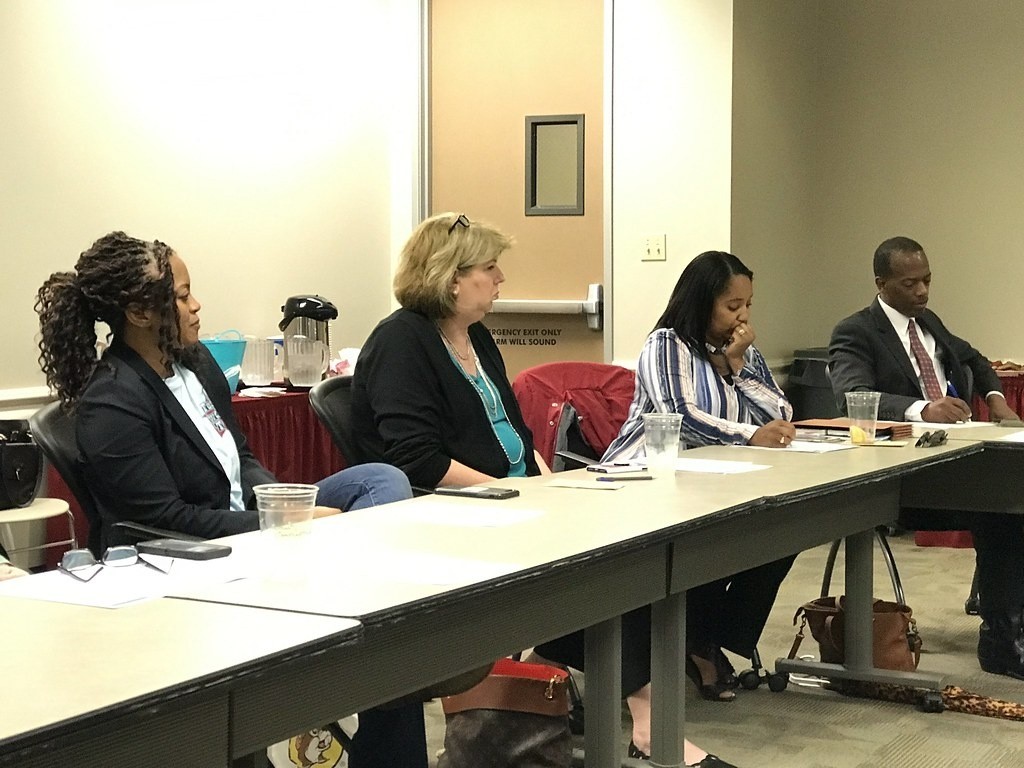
top-left (279, 293), bottom-right (338, 393)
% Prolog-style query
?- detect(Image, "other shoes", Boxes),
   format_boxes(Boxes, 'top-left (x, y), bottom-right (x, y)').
top-left (977, 634), bottom-right (1024, 681)
top-left (686, 653), bottom-right (736, 702)
top-left (628, 742), bottom-right (738, 768)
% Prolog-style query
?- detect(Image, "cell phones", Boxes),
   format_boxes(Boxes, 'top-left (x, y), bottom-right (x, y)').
top-left (136, 539), bottom-right (232, 561)
top-left (586, 462), bottom-right (647, 474)
top-left (434, 485), bottom-right (520, 500)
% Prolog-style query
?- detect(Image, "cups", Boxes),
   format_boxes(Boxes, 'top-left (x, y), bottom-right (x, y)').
top-left (845, 391), bottom-right (882, 444)
top-left (640, 413), bottom-right (685, 474)
top-left (286, 337), bottom-right (331, 387)
top-left (252, 483), bottom-right (320, 530)
top-left (238, 338), bottom-right (283, 386)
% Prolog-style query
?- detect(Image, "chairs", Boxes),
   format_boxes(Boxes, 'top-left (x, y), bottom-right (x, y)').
top-left (309, 374), bottom-right (584, 734)
top-left (513, 361), bottom-right (638, 475)
top-left (0, 498), bottom-right (77, 555)
top-left (29, 398), bottom-right (210, 561)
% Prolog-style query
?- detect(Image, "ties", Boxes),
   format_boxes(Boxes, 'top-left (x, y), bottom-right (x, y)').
top-left (909, 319), bottom-right (943, 402)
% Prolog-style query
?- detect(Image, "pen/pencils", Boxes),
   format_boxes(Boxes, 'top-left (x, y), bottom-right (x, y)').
top-left (947, 381), bottom-right (972, 425)
top-left (596, 475), bottom-right (652, 481)
top-left (780, 406), bottom-right (793, 450)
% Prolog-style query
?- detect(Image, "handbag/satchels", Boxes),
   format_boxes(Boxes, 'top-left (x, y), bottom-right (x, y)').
top-left (437, 657), bottom-right (577, 768)
top-left (787, 528), bottom-right (921, 671)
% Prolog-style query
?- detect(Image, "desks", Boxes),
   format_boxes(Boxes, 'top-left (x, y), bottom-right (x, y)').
top-left (50, 392), bottom-right (343, 549)
top-left (0, 421), bottom-right (1024, 768)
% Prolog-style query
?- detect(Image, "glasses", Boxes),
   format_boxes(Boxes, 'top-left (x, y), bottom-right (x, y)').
top-left (449, 215), bottom-right (471, 234)
top-left (915, 430), bottom-right (948, 447)
top-left (57, 545), bottom-right (174, 583)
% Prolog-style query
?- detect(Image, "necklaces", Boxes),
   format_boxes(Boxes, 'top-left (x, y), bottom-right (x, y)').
top-left (434, 319), bottom-right (524, 464)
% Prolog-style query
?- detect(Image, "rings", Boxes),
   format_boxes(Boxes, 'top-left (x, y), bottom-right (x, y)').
top-left (738, 329), bottom-right (746, 336)
top-left (780, 437), bottom-right (784, 443)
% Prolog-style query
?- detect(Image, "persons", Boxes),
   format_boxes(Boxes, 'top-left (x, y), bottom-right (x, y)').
top-left (829, 236), bottom-right (1024, 682)
top-left (33, 230), bottom-right (494, 768)
top-left (598, 251), bottom-right (802, 701)
top-left (352, 213), bottom-right (736, 768)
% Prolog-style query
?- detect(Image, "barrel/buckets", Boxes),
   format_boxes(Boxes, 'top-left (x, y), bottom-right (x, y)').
top-left (198, 330), bottom-right (248, 396)
